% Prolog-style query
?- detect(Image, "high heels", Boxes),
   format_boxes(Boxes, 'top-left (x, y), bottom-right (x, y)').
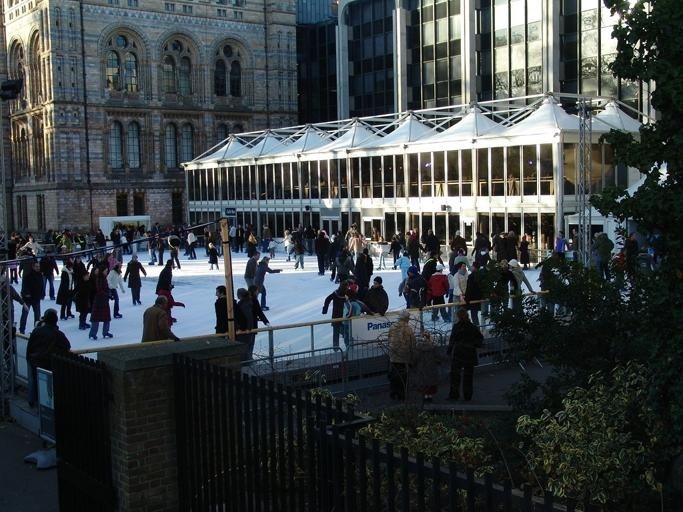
top-left (89, 334), bottom-right (97, 340)
top-left (66, 314), bottom-right (74, 318)
top-left (61, 316), bottom-right (67, 320)
top-left (103, 332), bottom-right (113, 337)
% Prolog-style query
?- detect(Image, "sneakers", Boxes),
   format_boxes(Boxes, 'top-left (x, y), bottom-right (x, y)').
top-left (114, 313), bottom-right (122, 318)
top-left (133, 301), bottom-right (136, 305)
top-left (269, 255), bottom-right (339, 284)
top-left (391, 267), bottom-right (396, 270)
top-left (138, 301), bottom-right (141, 304)
top-left (432, 316), bottom-right (439, 320)
top-left (443, 318), bottom-right (452, 322)
top-left (261, 306), bottom-right (269, 310)
top-left (382, 267), bottom-right (386, 269)
top-left (147, 257), bottom-right (220, 271)
top-left (522, 267), bottom-right (528, 270)
top-left (78, 324), bottom-right (91, 330)
top-left (11, 281), bottom-right (13, 283)
top-left (377, 267), bottom-right (381, 270)
top-left (15, 281), bottom-right (18, 284)
top-left (51, 296), bottom-right (55, 300)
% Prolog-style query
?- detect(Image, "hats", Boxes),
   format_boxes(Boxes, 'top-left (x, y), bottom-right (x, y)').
top-left (407, 266), bottom-right (418, 275)
top-left (42, 309), bottom-right (57, 318)
top-left (522, 235), bottom-right (526, 240)
top-left (374, 276), bottom-right (382, 284)
top-left (132, 253), bottom-right (138, 257)
top-left (436, 264), bottom-right (445, 270)
top-left (456, 230), bottom-right (460, 235)
top-left (397, 312), bottom-right (410, 320)
top-left (507, 259), bottom-right (518, 267)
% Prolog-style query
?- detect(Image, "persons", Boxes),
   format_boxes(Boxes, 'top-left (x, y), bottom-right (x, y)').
top-left (2, 223), bottom-right (662, 410)
